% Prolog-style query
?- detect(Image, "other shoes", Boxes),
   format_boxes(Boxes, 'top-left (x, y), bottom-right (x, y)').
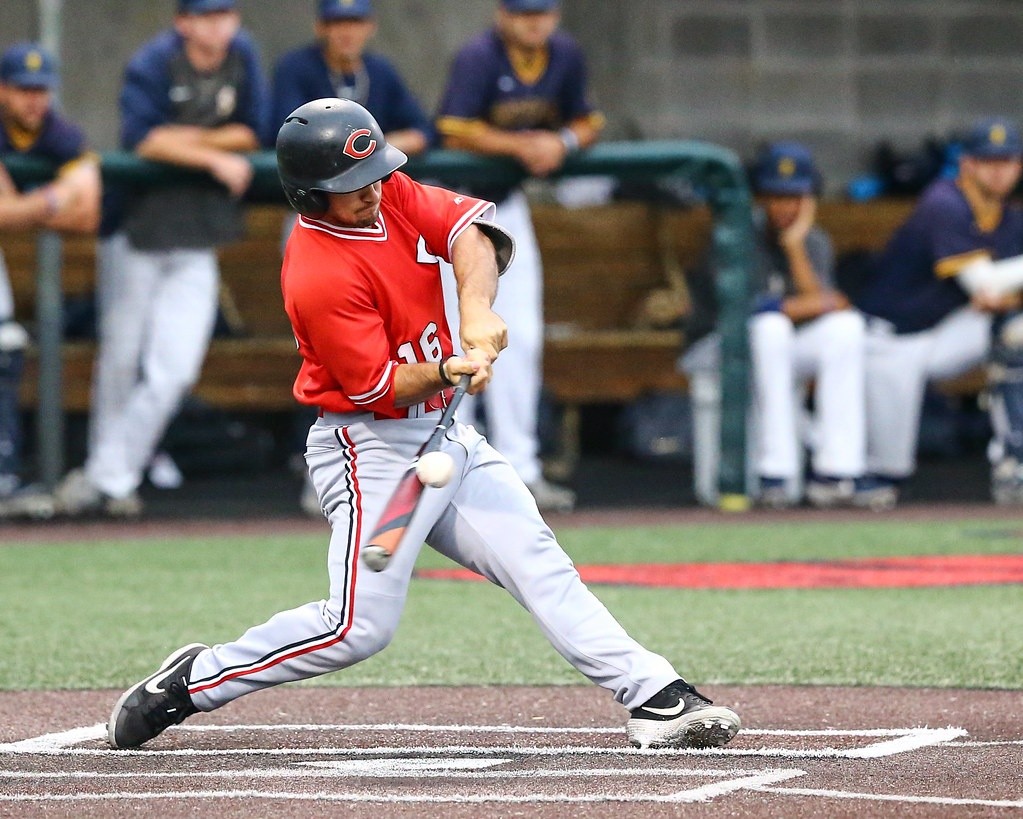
top-left (758, 472), bottom-right (900, 510)
top-left (1, 468), bottom-right (144, 517)
top-left (987, 461), bottom-right (1022, 507)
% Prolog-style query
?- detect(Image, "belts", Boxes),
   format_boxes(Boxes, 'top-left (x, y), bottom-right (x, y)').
top-left (372, 387), bottom-right (458, 419)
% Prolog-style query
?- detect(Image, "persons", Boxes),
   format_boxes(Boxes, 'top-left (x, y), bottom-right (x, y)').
top-left (107, 95), bottom-right (742, 752)
top-left (0, 0), bottom-right (271, 516)
top-left (269, 0), bottom-right (607, 175)
top-left (745, 115), bottom-right (1023, 509)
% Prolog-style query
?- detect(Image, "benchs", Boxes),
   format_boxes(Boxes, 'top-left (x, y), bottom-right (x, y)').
top-left (0, 200), bottom-right (990, 499)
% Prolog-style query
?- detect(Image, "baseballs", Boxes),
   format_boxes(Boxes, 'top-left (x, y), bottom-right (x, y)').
top-left (416, 452), bottom-right (454, 489)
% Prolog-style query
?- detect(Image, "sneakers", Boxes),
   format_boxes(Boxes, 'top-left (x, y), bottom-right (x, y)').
top-left (626, 679), bottom-right (742, 748)
top-left (105, 643), bottom-right (212, 749)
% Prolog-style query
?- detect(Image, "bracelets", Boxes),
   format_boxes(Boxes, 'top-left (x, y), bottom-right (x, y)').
top-left (439, 354), bottom-right (458, 387)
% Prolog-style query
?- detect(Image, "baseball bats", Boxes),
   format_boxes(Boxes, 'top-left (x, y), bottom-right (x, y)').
top-left (359, 373), bottom-right (472, 572)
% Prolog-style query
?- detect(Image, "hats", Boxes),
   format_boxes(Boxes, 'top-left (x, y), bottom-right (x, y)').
top-left (0, 41), bottom-right (59, 86)
top-left (955, 117), bottom-right (1023, 158)
top-left (312, 2), bottom-right (381, 22)
top-left (746, 142), bottom-right (821, 198)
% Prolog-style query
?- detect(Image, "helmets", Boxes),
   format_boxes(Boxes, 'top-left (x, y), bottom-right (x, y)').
top-left (276, 98), bottom-right (408, 221)
